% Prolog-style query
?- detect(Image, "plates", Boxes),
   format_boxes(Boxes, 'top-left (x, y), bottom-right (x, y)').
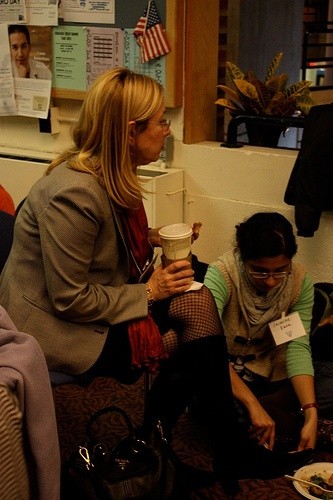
top-left (293, 463), bottom-right (333, 500)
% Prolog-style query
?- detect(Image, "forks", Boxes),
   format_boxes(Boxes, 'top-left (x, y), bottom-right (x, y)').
top-left (285, 475), bottom-right (333, 494)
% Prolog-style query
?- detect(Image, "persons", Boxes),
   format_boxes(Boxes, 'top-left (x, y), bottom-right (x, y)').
top-left (186, 212), bottom-right (317, 451)
top-left (0, 69), bottom-right (314, 500)
top-left (8, 25), bottom-right (52, 79)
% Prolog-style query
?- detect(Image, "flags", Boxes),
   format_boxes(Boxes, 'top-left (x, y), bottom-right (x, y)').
top-left (134, 0), bottom-right (172, 64)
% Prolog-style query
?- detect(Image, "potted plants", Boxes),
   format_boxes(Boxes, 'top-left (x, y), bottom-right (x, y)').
top-left (215, 51), bottom-right (315, 148)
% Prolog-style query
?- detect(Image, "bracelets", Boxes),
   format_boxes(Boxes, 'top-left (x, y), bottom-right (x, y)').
top-left (145, 283), bottom-right (154, 307)
top-left (300, 402), bottom-right (317, 414)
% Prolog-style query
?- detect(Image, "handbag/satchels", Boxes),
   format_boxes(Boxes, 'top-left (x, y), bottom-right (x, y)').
top-left (60, 405), bottom-right (177, 500)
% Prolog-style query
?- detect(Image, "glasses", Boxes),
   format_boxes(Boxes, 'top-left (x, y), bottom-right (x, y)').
top-left (142, 119), bottom-right (172, 132)
top-left (241, 255), bottom-right (292, 280)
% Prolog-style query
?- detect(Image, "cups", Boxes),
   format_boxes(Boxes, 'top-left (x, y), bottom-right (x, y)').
top-left (159, 224), bottom-right (192, 260)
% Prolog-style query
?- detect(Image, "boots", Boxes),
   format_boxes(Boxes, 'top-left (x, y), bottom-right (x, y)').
top-left (180, 334), bottom-right (285, 496)
top-left (132, 349), bottom-right (219, 500)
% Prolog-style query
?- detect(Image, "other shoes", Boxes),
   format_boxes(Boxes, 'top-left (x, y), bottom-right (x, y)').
top-left (293, 413), bottom-right (333, 448)
